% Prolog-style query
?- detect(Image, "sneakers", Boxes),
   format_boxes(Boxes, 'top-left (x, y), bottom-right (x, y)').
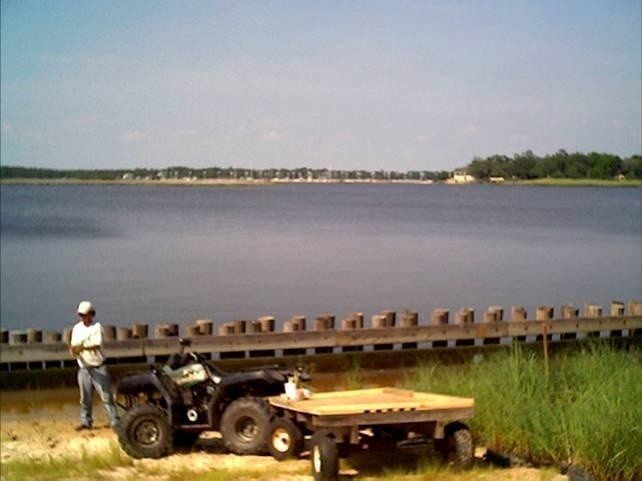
top-left (74, 422), bottom-right (92, 431)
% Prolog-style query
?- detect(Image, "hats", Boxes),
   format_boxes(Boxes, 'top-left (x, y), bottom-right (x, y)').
top-left (77, 301), bottom-right (95, 316)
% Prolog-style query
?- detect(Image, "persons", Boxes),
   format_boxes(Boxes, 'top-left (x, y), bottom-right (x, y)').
top-left (68, 300), bottom-right (120, 432)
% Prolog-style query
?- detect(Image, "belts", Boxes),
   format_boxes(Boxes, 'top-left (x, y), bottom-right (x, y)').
top-left (84, 364), bottom-right (103, 368)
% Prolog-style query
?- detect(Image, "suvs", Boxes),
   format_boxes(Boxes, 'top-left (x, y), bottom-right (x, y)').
top-left (114, 336), bottom-right (312, 459)
